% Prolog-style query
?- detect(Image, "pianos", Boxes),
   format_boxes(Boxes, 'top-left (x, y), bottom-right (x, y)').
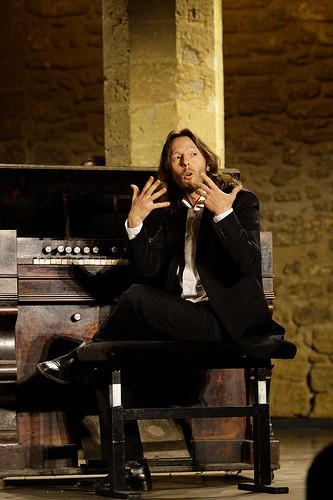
top-left (1, 162), bottom-right (282, 484)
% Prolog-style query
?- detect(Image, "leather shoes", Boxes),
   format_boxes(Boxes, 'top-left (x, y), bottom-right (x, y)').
top-left (37, 341), bottom-right (107, 386)
top-left (95, 461), bottom-right (153, 493)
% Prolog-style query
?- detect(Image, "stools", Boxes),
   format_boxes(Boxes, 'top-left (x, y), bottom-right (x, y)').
top-left (74, 336), bottom-right (298, 500)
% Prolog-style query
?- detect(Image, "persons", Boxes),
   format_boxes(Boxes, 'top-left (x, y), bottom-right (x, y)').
top-left (35, 128), bottom-right (285, 499)
